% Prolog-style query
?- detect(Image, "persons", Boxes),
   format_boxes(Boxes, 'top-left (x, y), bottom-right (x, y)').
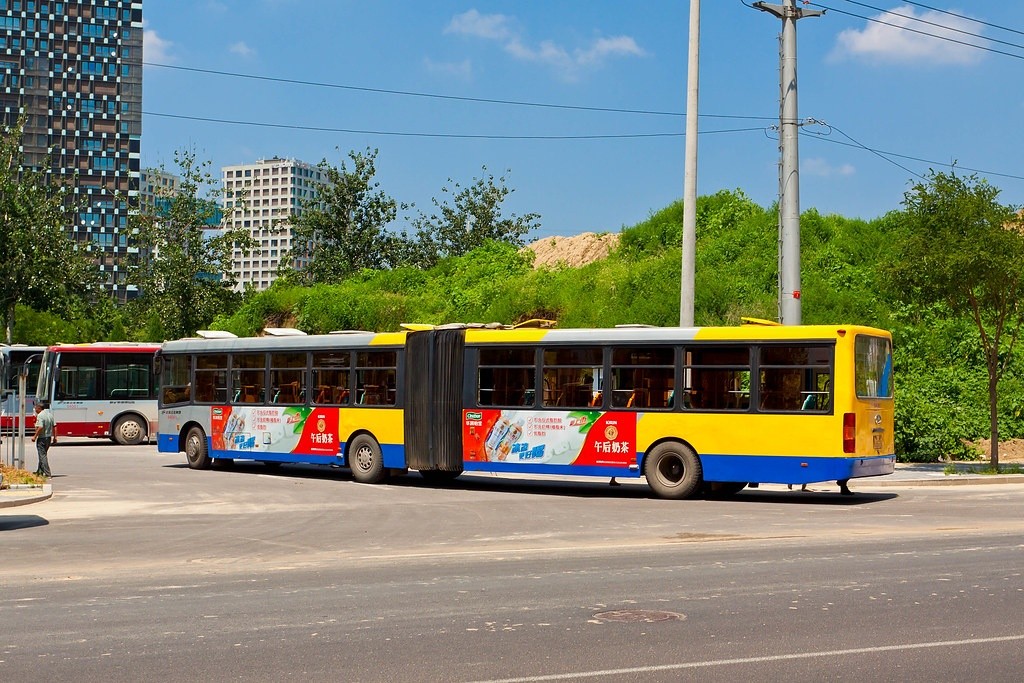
top-left (788, 483), bottom-right (806, 491)
top-left (837, 478), bottom-right (854, 495)
top-left (31, 403), bottom-right (57, 478)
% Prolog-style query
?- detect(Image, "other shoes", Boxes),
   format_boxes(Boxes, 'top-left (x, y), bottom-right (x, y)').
top-left (46, 474), bottom-right (52, 478)
top-left (33, 472), bottom-right (43, 476)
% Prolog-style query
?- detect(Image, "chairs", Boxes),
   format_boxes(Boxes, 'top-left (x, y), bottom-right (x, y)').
top-left (189, 382), bottom-right (379, 406)
top-left (557, 390), bottom-right (692, 409)
top-left (760, 394), bottom-right (817, 411)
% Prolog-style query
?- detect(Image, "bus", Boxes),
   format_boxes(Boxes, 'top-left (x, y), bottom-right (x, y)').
top-left (0, 340), bottom-right (169, 446)
top-left (152, 315), bottom-right (897, 501)
